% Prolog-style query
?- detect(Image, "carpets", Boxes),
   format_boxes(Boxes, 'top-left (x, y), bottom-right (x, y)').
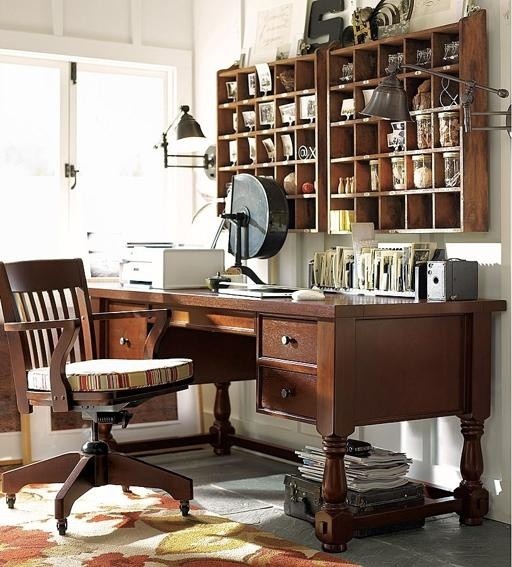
top-left (0, 467), bottom-right (360, 567)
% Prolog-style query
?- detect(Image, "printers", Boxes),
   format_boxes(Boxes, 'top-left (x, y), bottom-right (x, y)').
top-left (119, 247), bottom-right (224, 289)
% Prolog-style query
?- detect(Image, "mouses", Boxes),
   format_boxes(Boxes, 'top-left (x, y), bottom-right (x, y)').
top-left (292, 290), bottom-right (325, 301)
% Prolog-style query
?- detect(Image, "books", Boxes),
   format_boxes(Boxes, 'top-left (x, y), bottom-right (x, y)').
top-left (312, 222), bottom-right (444, 292)
top-left (295, 443), bottom-right (414, 490)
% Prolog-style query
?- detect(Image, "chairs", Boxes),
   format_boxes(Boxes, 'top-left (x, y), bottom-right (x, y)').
top-left (0, 257), bottom-right (195, 537)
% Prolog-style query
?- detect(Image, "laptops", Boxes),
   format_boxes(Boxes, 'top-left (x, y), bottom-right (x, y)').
top-left (218, 282), bottom-right (323, 299)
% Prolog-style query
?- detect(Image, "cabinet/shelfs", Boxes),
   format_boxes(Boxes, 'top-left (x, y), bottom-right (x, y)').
top-left (325, 7), bottom-right (490, 234)
top-left (215, 47), bottom-right (322, 234)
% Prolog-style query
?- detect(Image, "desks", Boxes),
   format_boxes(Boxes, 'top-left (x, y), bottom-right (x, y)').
top-left (85, 283), bottom-right (509, 554)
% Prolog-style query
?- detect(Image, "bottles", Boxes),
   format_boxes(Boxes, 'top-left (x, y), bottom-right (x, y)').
top-left (337, 176), bottom-right (354, 194)
top-left (369, 110), bottom-right (463, 190)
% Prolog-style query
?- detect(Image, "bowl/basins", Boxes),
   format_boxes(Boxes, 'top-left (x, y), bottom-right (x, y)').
top-left (205, 271), bottom-right (232, 291)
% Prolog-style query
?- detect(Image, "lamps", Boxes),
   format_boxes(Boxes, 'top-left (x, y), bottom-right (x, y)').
top-left (359, 59), bottom-right (512, 142)
top-left (154, 106), bottom-right (217, 182)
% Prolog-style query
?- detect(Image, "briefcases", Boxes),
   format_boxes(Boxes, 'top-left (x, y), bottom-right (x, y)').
top-left (283, 469), bottom-right (427, 538)
